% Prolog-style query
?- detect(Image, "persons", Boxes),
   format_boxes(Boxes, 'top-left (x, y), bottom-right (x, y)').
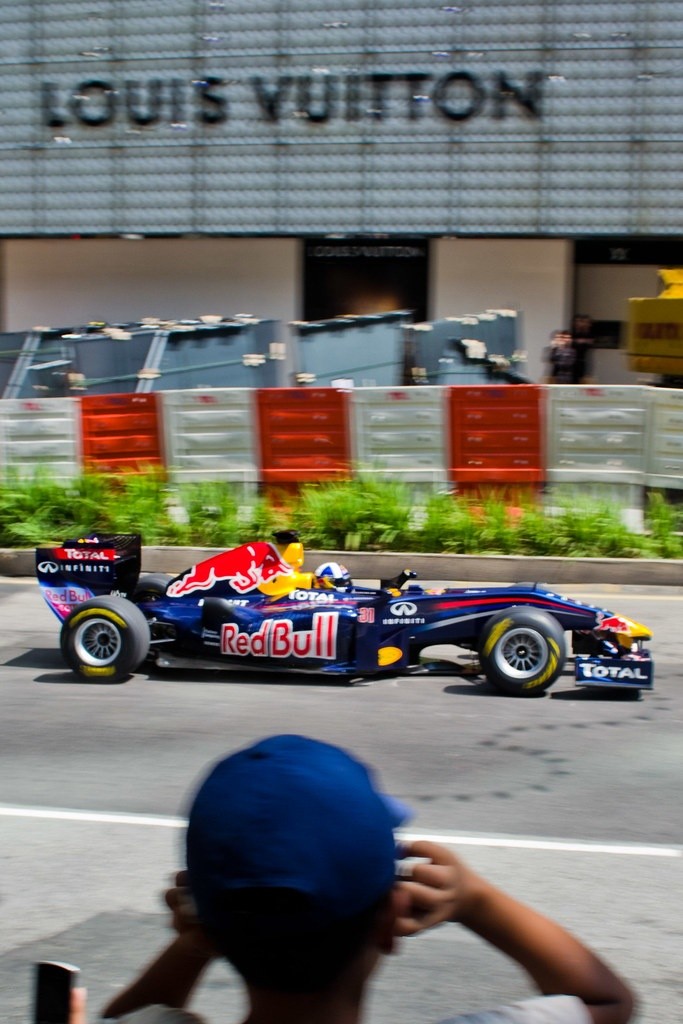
top-left (541, 314), bottom-right (597, 385)
top-left (314, 562), bottom-right (357, 594)
top-left (89, 735), bottom-right (634, 1024)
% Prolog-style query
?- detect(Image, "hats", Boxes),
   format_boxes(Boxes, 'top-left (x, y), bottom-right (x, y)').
top-left (185, 734), bottom-right (410, 923)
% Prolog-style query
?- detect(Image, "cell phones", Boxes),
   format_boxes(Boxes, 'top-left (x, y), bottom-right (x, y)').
top-left (34, 961), bottom-right (81, 1024)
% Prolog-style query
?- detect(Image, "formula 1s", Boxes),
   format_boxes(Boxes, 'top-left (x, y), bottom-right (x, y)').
top-left (34, 529), bottom-right (656, 697)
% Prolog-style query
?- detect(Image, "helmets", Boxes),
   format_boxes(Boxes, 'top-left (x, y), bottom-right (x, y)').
top-left (314, 561), bottom-right (353, 594)
